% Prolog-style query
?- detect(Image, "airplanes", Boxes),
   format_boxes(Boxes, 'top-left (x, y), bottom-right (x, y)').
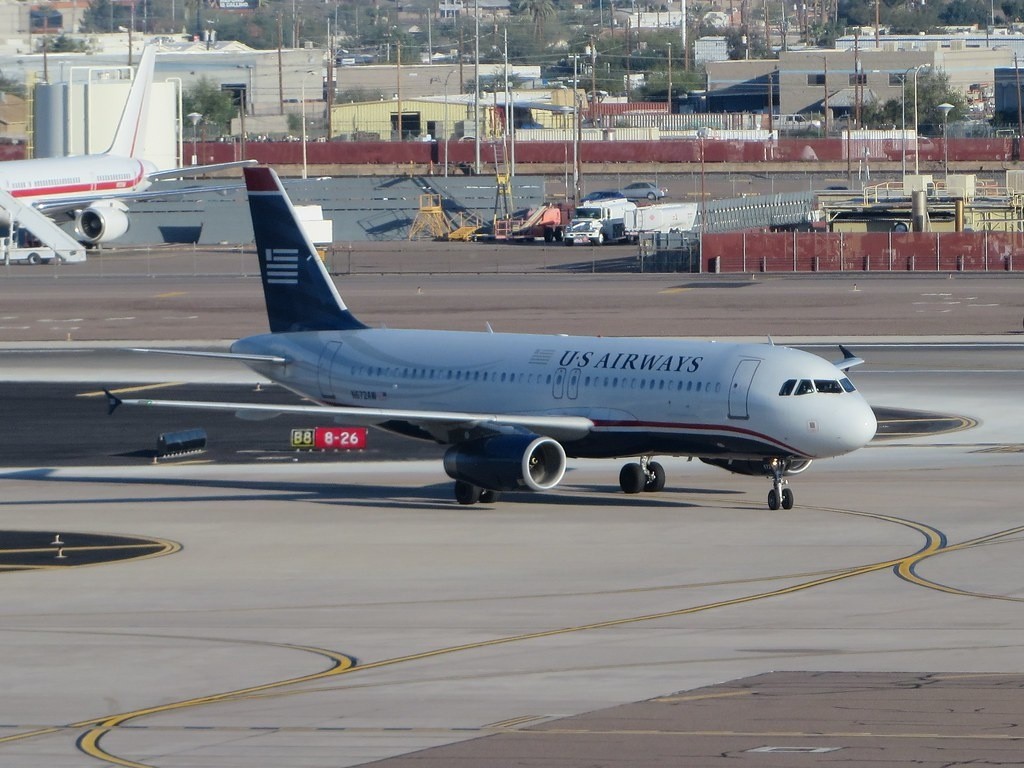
top-left (0, 43), bottom-right (259, 266)
top-left (0, 166), bottom-right (877, 511)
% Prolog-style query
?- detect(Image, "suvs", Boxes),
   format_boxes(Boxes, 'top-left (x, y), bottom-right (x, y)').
top-left (563, 218), bottom-right (604, 246)
top-left (771, 115), bottom-right (817, 132)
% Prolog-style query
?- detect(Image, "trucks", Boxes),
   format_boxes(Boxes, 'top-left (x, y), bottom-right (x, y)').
top-left (576, 198), bottom-right (635, 243)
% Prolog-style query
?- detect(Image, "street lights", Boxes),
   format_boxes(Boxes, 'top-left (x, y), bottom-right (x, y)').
top-left (568, 53), bottom-right (580, 182)
top-left (559, 105), bottom-right (574, 202)
top-left (902, 66), bottom-right (918, 178)
top-left (302, 71), bottom-right (315, 179)
top-left (327, 47), bottom-right (349, 140)
top-left (444, 69), bottom-right (456, 177)
top-left (937, 101), bottom-right (954, 182)
top-left (185, 111), bottom-right (203, 179)
top-left (914, 64), bottom-right (930, 174)
top-left (696, 126), bottom-right (709, 233)
top-left (492, 44), bottom-right (508, 141)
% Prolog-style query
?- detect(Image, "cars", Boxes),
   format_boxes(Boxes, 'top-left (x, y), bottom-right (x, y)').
top-left (580, 189), bottom-right (624, 201)
top-left (586, 90), bottom-right (607, 102)
top-left (621, 181), bottom-right (666, 200)
